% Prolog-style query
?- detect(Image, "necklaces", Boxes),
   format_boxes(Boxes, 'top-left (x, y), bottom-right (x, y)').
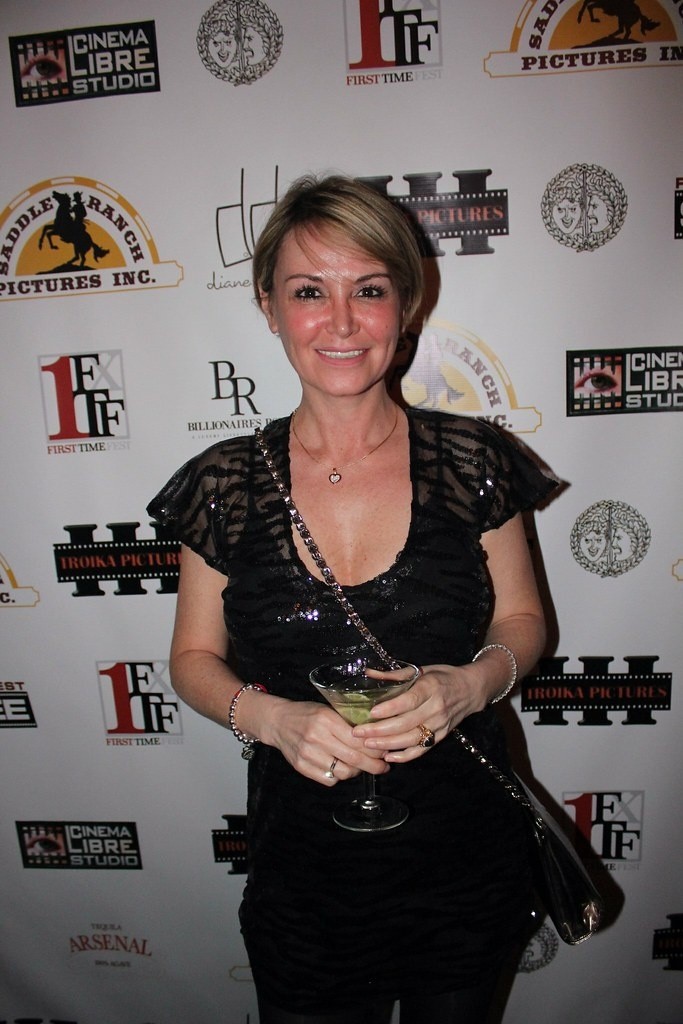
top-left (292, 401), bottom-right (398, 484)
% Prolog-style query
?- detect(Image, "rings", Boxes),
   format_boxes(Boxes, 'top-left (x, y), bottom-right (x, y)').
top-left (416, 724), bottom-right (434, 747)
top-left (325, 756), bottom-right (338, 779)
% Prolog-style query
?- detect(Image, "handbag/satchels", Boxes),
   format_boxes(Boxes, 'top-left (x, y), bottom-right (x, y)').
top-left (502, 760), bottom-right (626, 945)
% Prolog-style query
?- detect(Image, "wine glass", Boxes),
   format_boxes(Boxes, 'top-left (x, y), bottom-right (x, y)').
top-left (308, 658), bottom-right (421, 830)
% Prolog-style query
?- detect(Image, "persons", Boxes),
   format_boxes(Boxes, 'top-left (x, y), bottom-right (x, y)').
top-left (145, 176), bottom-right (559, 1023)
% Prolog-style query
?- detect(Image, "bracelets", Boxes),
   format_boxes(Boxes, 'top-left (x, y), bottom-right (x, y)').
top-left (229, 682), bottom-right (267, 760)
top-left (472, 644), bottom-right (517, 705)
